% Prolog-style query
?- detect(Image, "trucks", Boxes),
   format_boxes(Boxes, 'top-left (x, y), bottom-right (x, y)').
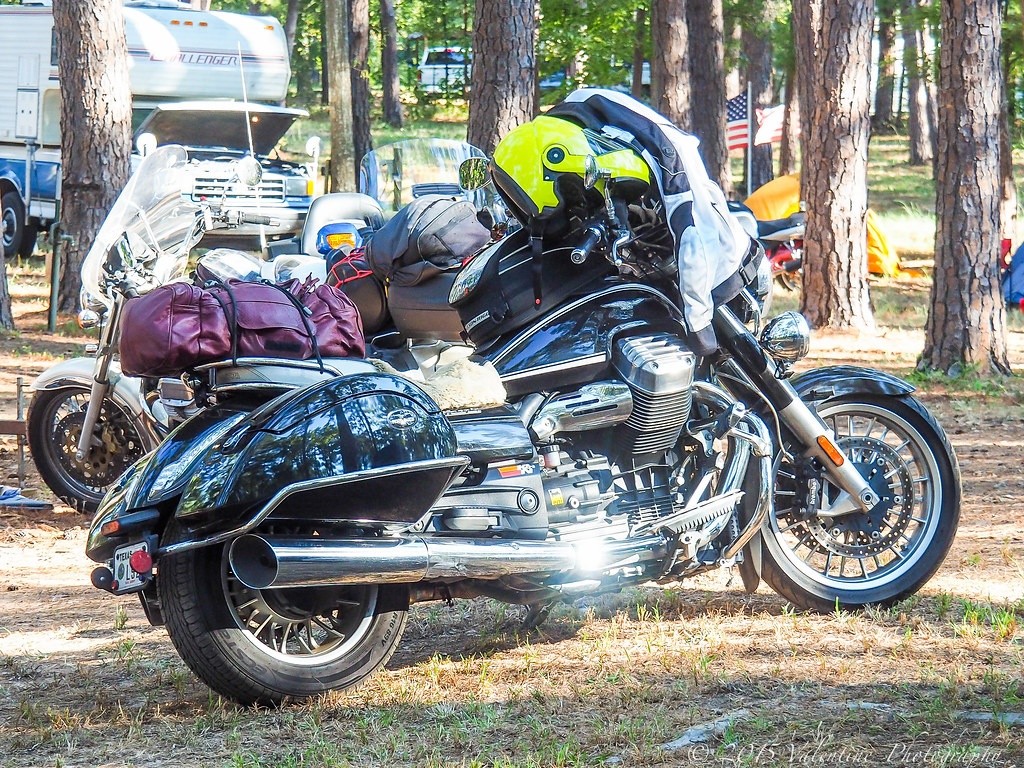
top-left (0, 1), bottom-right (322, 259)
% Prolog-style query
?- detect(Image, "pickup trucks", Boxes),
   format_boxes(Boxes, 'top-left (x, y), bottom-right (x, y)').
top-left (418, 46), bottom-right (474, 96)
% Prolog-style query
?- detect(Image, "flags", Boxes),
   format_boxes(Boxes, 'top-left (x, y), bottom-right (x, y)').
top-left (725, 89), bottom-right (748, 150)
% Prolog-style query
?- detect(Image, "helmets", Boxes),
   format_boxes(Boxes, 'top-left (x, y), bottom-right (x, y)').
top-left (491, 115), bottom-right (651, 236)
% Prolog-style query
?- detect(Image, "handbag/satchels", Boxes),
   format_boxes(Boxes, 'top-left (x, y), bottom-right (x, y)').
top-left (118, 272), bottom-right (365, 378)
top-left (365, 194), bottom-right (491, 287)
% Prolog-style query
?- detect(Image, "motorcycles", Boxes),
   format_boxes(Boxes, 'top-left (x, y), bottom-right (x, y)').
top-left (25, 124), bottom-right (533, 519)
top-left (88, 85), bottom-right (963, 717)
